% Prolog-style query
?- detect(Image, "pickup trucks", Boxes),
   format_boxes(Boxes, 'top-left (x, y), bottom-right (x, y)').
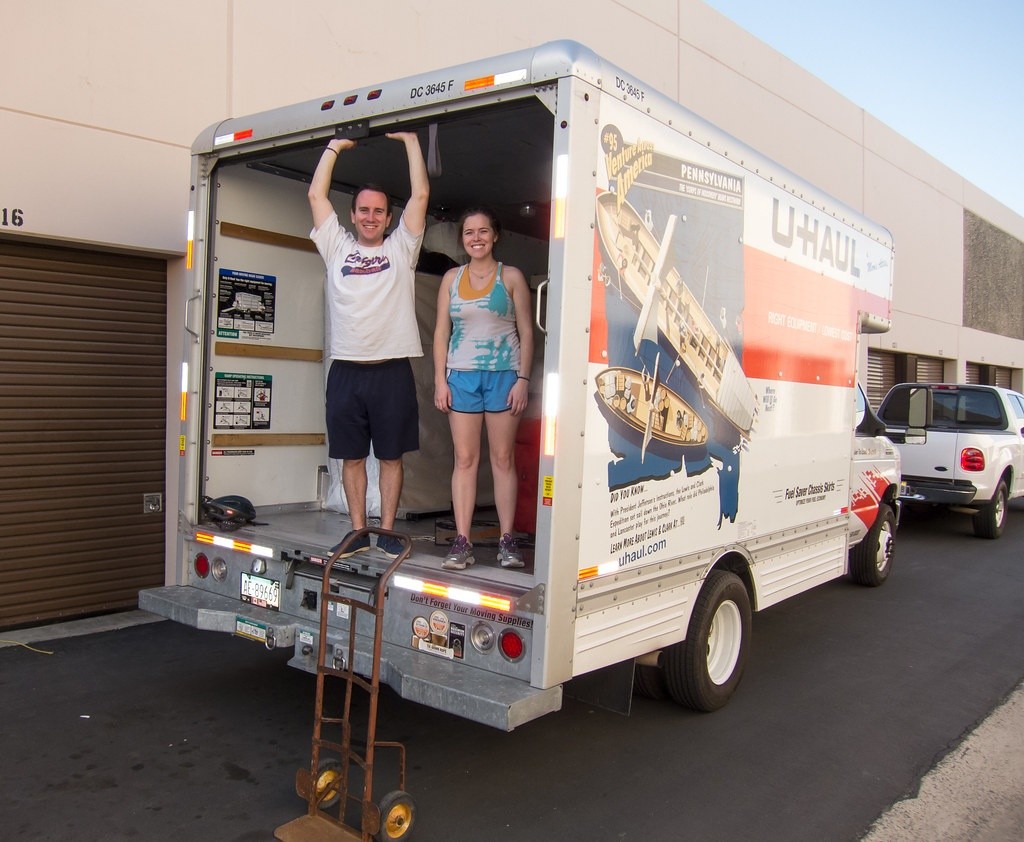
top-left (875, 383), bottom-right (1024, 538)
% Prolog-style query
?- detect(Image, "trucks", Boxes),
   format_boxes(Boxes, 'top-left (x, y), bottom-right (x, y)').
top-left (135, 39), bottom-right (934, 734)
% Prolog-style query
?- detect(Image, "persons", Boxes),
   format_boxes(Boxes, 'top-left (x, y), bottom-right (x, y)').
top-left (433, 203), bottom-right (534, 570)
top-left (308, 131), bottom-right (429, 559)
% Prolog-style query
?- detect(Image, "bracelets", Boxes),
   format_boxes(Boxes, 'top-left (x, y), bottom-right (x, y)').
top-left (326, 146), bottom-right (339, 158)
top-left (517, 377), bottom-right (530, 382)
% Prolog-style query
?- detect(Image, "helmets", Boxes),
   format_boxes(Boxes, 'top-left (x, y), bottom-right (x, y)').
top-left (203, 496), bottom-right (256, 530)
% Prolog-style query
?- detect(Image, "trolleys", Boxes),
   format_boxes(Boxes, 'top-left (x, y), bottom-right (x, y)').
top-left (271, 527), bottom-right (417, 842)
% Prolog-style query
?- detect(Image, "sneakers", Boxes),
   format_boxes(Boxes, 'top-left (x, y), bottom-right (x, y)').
top-left (376, 535), bottom-right (409, 559)
top-left (441, 534), bottom-right (475, 569)
top-left (327, 531), bottom-right (371, 558)
top-left (496, 534), bottom-right (525, 568)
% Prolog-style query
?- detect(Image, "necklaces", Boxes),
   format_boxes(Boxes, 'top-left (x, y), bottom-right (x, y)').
top-left (470, 260), bottom-right (497, 279)
